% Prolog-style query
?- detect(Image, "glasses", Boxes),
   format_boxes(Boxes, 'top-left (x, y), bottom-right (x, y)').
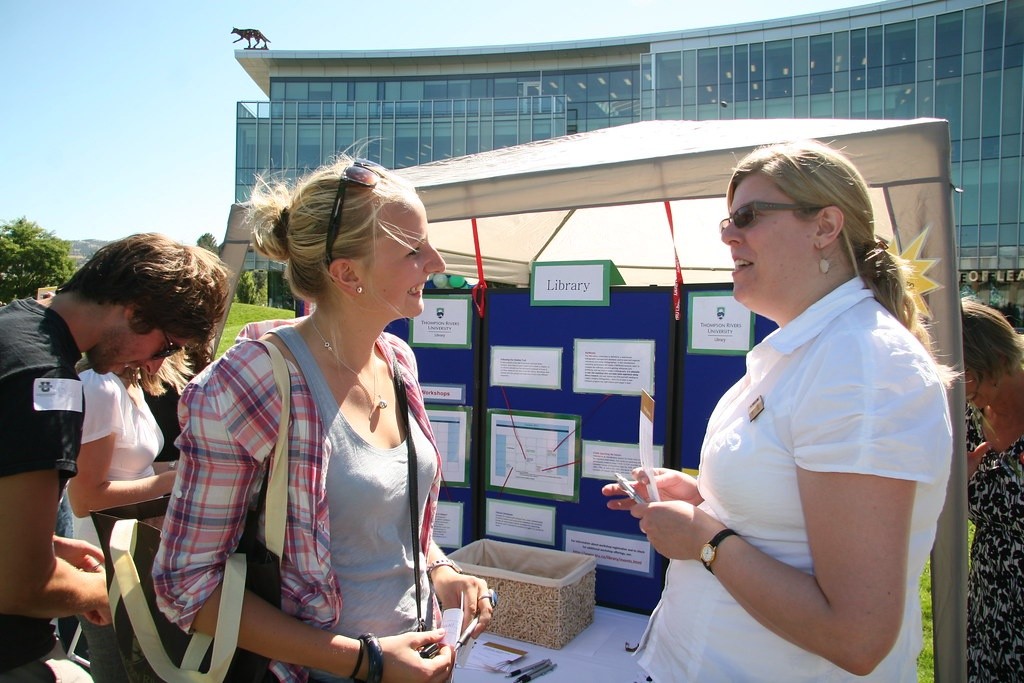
top-left (323, 155), bottom-right (390, 283)
top-left (151, 324), bottom-right (182, 360)
top-left (718, 200), bottom-right (818, 235)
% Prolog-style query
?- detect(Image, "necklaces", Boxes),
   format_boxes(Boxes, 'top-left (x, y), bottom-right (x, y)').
top-left (310, 312), bottom-right (390, 410)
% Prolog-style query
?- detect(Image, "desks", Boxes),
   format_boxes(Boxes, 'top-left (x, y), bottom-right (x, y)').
top-left (430, 584), bottom-right (653, 683)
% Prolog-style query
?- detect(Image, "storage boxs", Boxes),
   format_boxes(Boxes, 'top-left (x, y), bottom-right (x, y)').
top-left (444, 539), bottom-right (598, 651)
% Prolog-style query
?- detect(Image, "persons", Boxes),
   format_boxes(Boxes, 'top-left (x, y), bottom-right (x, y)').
top-left (65, 346), bottom-right (198, 683)
top-left (602, 139), bottom-right (954, 683)
top-left (150, 153), bottom-right (494, 683)
top-left (961, 296), bottom-right (1024, 683)
top-left (0, 232), bottom-right (226, 683)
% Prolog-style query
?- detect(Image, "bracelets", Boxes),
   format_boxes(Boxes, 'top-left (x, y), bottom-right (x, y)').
top-left (349, 638), bottom-right (365, 678)
top-left (360, 633), bottom-right (383, 683)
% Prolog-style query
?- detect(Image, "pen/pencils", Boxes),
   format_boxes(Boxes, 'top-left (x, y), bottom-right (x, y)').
top-left (453, 616), bottom-right (479, 652)
top-left (614, 473), bottom-right (646, 506)
top-left (517, 663), bottom-right (557, 683)
top-left (505, 659), bottom-right (546, 677)
top-left (512, 661), bottom-right (552, 683)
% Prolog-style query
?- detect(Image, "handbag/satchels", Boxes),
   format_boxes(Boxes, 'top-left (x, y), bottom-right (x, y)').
top-left (88, 339), bottom-right (291, 682)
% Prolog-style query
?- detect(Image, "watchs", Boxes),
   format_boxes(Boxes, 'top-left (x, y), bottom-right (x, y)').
top-left (701, 528), bottom-right (740, 575)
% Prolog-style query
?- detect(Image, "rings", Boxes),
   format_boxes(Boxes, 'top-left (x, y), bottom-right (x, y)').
top-left (477, 589), bottom-right (499, 607)
